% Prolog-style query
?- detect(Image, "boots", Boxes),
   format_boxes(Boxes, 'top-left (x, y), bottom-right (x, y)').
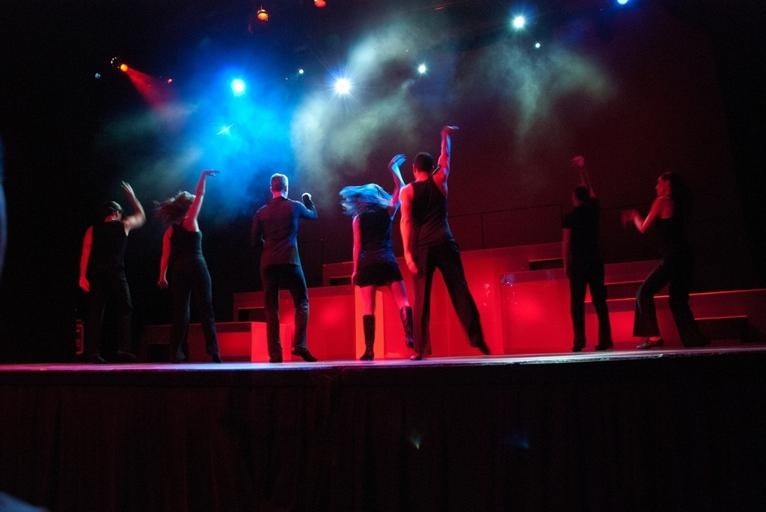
top-left (358, 315), bottom-right (375, 360)
top-left (400, 306), bottom-right (417, 347)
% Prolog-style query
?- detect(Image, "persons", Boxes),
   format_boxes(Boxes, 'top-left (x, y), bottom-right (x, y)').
top-left (399, 125), bottom-right (492, 360)
top-left (561, 155), bottom-right (613, 351)
top-left (251, 173), bottom-right (319, 363)
top-left (339, 152), bottom-right (416, 361)
top-left (151, 170), bottom-right (227, 363)
top-left (619, 170), bottom-right (711, 349)
top-left (76, 181), bottom-right (148, 364)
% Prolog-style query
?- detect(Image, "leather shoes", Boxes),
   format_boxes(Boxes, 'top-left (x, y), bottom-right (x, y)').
top-left (636, 338), bottom-right (664, 350)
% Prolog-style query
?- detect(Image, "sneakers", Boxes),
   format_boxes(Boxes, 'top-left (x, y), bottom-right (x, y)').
top-left (290, 346), bottom-right (317, 362)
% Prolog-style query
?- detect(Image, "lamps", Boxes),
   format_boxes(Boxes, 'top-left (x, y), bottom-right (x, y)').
top-left (106, 52), bottom-right (130, 74)
top-left (246, 1), bottom-right (269, 35)
